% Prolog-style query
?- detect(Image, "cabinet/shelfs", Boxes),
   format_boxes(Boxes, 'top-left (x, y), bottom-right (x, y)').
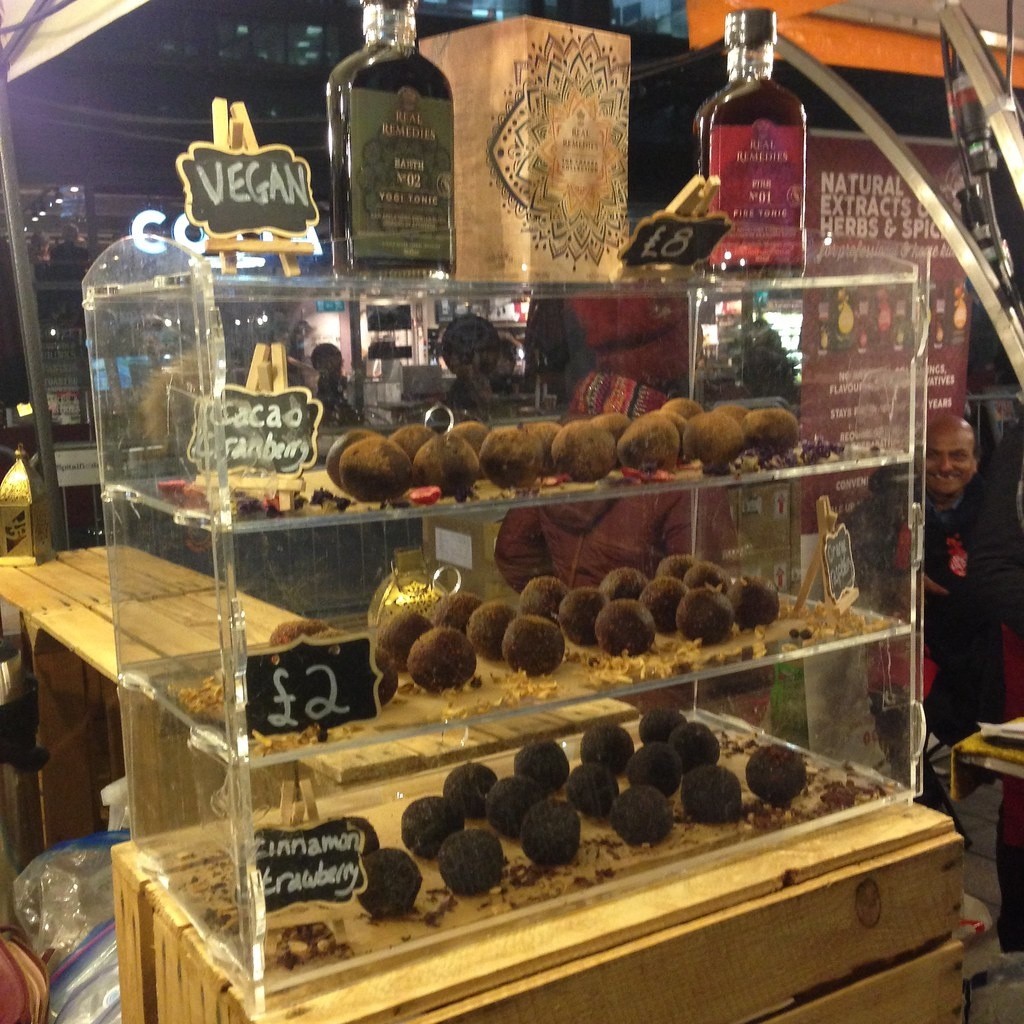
top-left (80, 229), bottom-right (931, 1015)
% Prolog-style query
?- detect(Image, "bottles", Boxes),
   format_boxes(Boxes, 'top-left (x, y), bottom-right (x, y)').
top-left (691, 8), bottom-right (808, 274)
top-left (332, 0), bottom-right (457, 280)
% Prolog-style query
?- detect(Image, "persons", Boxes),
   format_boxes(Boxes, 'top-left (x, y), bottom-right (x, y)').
top-left (440, 279), bottom-right (803, 729)
top-left (840, 416), bottom-right (1024, 953)
top-left (28, 225), bottom-right (127, 327)
top-left (233, 321), bottom-right (362, 428)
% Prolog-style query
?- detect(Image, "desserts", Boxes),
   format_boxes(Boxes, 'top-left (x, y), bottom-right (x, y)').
top-left (273, 397), bottom-right (806, 914)
